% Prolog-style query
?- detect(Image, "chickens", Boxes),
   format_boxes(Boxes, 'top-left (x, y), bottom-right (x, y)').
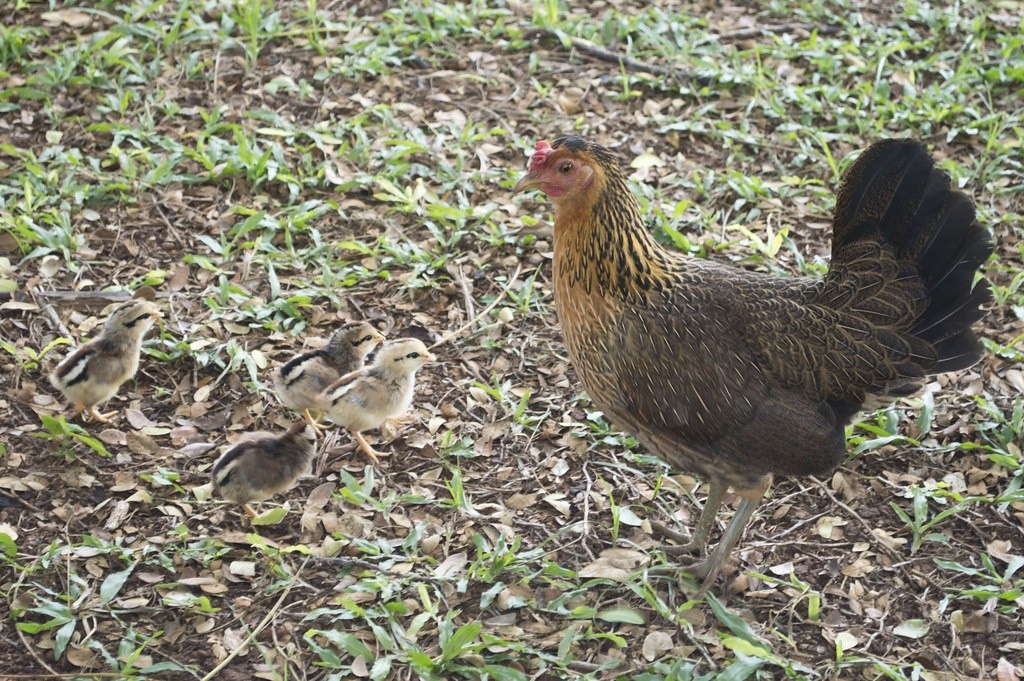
top-left (47, 299), bottom-right (166, 428)
top-left (274, 321), bottom-right (436, 465)
top-left (513, 136), bottom-right (995, 603)
top-left (211, 421), bottom-right (318, 518)
